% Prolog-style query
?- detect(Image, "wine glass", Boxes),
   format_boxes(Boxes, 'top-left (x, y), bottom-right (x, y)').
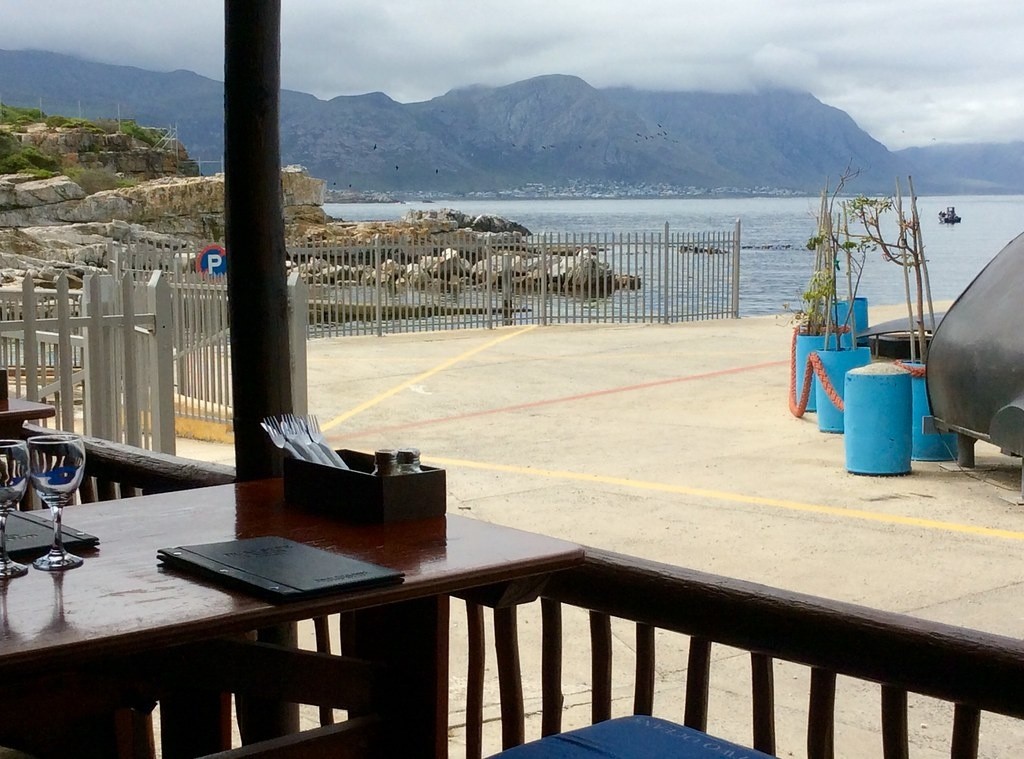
top-left (28, 435), bottom-right (86, 570)
top-left (0, 439), bottom-right (30, 578)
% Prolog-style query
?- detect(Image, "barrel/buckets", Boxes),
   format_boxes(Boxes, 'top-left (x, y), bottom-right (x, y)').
top-left (845, 297), bottom-right (868, 332)
top-left (909, 366), bottom-right (957, 460)
top-left (816, 347), bottom-right (870, 433)
top-left (820, 302), bottom-right (852, 350)
top-left (796, 333), bottom-right (844, 410)
top-left (844, 373), bottom-right (913, 474)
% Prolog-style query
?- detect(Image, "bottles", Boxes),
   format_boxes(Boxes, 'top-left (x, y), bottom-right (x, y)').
top-left (398, 448), bottom-right (423, 474)
top-left (371, 449), bottom-right (401, 476)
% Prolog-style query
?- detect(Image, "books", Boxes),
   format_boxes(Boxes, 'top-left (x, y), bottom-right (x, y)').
top-left (0, 510), bottom-right (100, 561)
top-left (157, 536), bottom-right (406, 606)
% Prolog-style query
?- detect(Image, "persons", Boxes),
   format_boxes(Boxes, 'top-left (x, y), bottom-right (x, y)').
top-left (939, 208), bottom-right (955, 218)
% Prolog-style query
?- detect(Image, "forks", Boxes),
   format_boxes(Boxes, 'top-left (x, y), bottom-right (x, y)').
top-left (263, 415), bottom-right (349, 470)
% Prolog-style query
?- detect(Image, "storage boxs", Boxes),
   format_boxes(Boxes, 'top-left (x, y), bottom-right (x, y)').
top-left (282, 448), bottom-right (448, 524)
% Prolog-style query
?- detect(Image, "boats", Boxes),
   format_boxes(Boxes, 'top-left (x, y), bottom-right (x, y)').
top-left (938, 206), bottom-right (961, 223)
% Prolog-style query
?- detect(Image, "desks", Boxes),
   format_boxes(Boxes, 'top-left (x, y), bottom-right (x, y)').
top-left (0, 398), bottom-right (56, 511)
top-left (0, 478), bottom-right (583, 759)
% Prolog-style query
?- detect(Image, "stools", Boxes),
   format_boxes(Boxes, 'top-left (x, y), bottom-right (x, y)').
top-left (483, 714), bottom-right (783, 759)
